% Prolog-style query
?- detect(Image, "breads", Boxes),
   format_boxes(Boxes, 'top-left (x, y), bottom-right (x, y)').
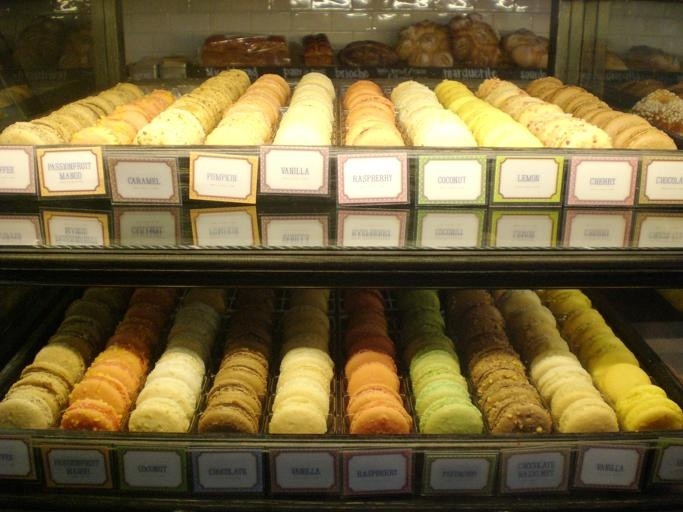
top-left (128, 11), bottom-right (683, 68)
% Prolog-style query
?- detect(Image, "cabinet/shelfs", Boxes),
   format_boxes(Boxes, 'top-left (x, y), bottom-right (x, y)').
top-left (0, 0), bottom-right (682, 510)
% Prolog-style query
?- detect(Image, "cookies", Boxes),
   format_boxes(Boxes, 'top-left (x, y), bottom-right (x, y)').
top-left (0, 284), bottom-right (683, 434)
top-left (0, 69), bottom-right (683, 205)
